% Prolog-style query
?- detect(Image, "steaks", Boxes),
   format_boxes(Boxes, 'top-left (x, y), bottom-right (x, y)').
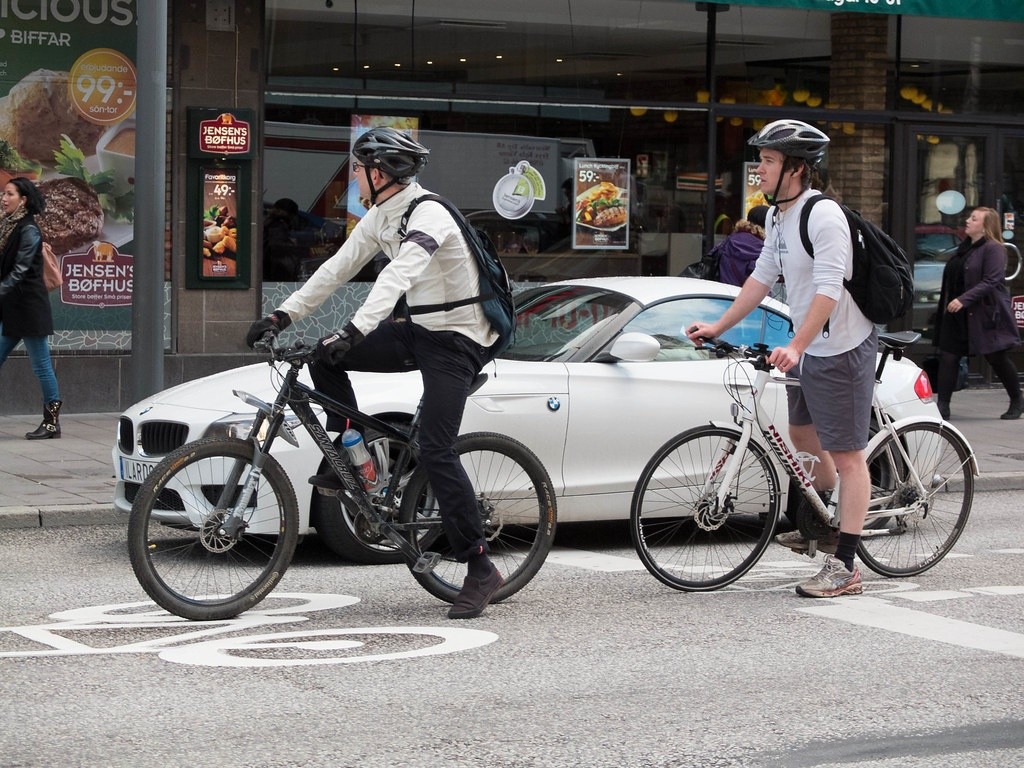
top-left (22, 176), bottom-right (104, 250)
top-left (592, 206), bottom-right (626, 226)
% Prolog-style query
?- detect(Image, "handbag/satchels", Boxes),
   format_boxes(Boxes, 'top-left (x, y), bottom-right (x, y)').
top-left (41, 241), bottom-right (64, 292)
top-left (677, 241), bottom-right (728, 280)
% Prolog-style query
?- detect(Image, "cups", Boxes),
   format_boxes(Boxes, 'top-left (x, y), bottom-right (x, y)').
top-left (342, 430), bottom-right (383, 493)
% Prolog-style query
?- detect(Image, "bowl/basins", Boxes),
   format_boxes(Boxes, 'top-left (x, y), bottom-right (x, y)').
top-left (96, 118), bottom-right (135, 182)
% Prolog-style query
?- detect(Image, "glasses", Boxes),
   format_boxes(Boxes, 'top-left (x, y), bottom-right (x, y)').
top-left (353, 161), bottom-right (373, 172)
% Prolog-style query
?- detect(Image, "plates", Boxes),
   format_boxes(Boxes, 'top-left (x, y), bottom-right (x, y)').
top-left (0, 96), bottom-right (134, 265)
top-left (576, 182), bottom-right (627, 232)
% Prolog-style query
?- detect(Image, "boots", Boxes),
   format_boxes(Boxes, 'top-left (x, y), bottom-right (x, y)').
top-left (25, 400), bottom-right (63, 439)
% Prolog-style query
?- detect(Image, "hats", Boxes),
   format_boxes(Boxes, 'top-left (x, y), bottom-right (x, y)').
top-left (747, 205), bottom-right (770, 228)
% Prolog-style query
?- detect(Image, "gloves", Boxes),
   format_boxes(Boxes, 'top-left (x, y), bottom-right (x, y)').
top-left (246, 310), bottom-right (292, 351)
top-left (316, 321), bottom-right (365, 366)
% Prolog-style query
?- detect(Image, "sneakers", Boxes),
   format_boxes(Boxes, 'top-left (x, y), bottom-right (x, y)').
top-left (448, 564), bottom-right (505, 619)
top-left (775, 529), bottom-right (840, 554)
top-left (795, 554), bottom-right (863, 598)
top-left (308, 462), bottom-right (362, 490)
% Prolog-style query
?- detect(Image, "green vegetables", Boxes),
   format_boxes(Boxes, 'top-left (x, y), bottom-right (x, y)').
top-left (204, 205), bottom-right (221, 221)
top-left (576, 199), bottom-right (620, 221)
top-left (0, 140), bottom-right (137, 224)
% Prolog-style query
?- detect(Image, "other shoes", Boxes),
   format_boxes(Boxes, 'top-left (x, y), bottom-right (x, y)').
top-left (1001, 392), bottom-right (1024, 419)
top-left (937, 401), bottom-right (950, 420)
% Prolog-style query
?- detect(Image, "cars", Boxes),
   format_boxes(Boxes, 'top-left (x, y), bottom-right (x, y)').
top-left (112, 278), bottom-right (944, 564)
top-left (914, 223), bottom-right (970, 305)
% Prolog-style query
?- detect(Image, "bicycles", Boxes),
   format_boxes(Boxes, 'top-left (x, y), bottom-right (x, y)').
top-left (631, 327), bottom-right (978, 594)
top-left (125, 331), bottom-right (559, 622)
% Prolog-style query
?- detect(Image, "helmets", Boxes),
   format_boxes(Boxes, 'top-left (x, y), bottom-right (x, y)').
top-left (747, 119), bottom-right (830, 158)
top-left (352, 126), bottom-right (432, 178)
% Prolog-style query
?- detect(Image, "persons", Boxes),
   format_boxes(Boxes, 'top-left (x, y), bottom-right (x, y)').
top-left (0, 176), bottom-right (64, 439)
top-left (701, 207), bottom-right (770, 285)
top-left (684, 120), bottom-right (878, 599)
top-left (245, 126), bottom-right (508, 620)
top-left (932, 206), bottom-right (1024, 420)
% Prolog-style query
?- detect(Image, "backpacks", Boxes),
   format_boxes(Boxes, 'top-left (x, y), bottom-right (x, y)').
top-left (800, 195), bottom-right (915, 325)
top-left (398, 194), bottom-right (517, 363)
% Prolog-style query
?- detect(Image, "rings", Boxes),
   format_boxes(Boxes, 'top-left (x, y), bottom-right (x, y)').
top-left (780, 364), bottom-right (784, 369)
